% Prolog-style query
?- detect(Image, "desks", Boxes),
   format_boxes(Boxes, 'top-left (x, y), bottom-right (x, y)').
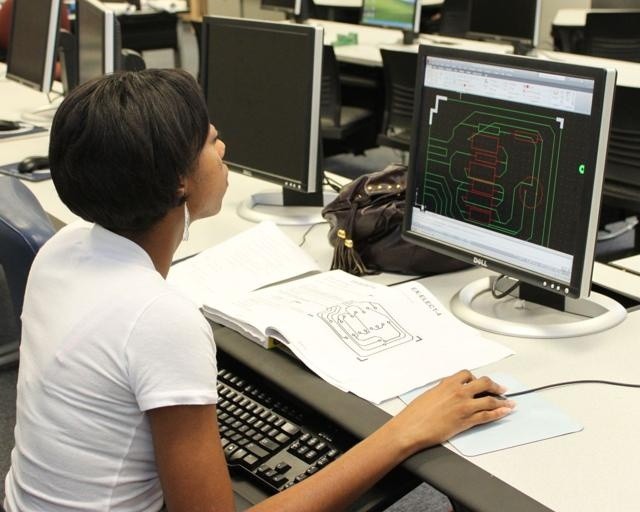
top-left (4, 17), bottom-right (640, 510)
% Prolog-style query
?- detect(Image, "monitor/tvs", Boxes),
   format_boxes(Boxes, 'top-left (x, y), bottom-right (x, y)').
top-left (360, 0), bottom-right (431, 52)
top-left (400, 44), bottom-right (627, 338)
top-left (259, 0), bottom-right (322, 24)
top-left (72, 0), bottom-right (121, 100)
top-left (197, 15), bottom-right (346, 225)
top-left (465, 0), bottom-right (556, 61)
top-left (5, 0), bottom-right (72, 123)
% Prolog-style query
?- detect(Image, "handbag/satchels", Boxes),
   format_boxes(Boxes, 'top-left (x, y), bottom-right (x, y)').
top-left (321, 164), bottom-right (475, 277)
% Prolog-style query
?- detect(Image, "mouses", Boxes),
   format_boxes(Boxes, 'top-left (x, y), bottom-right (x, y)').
top-left (0, 119), bottom-right (20, 130)
top-left (18, 156), bottom-right (50, 173)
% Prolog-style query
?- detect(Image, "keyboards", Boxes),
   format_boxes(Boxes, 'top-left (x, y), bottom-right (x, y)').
top-left (215, 361), bottom-right (343, 494)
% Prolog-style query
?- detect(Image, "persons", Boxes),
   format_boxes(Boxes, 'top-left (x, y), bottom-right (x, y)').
top-left (3, 68), bottom-right (516, 512)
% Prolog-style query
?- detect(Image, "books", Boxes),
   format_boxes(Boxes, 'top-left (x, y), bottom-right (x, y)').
top-left (202, 268), bottom-right (515, 406)
